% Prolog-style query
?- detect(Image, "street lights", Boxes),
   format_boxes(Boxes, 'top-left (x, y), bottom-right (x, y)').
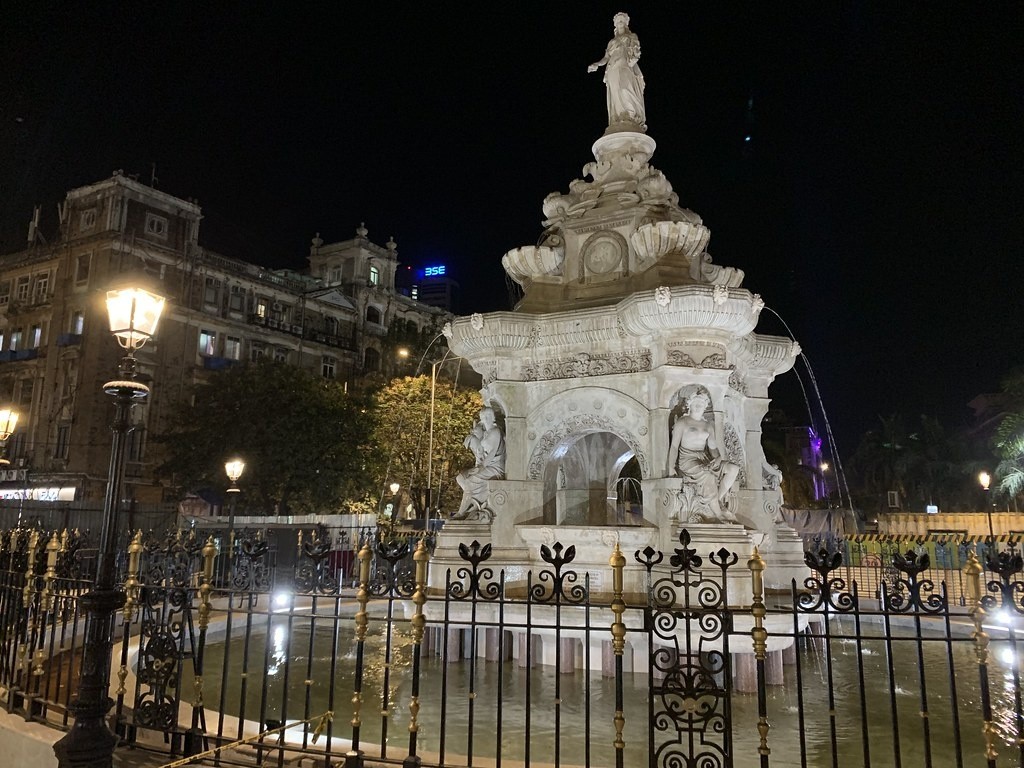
top-left (222, 456), bottom-right (246, 589)
top-left (388, 480), bottom-right (401, 532)
top-left (48, 270), bottom-right (170, 765)
top-left (977, 472), bottom-right (995, 550)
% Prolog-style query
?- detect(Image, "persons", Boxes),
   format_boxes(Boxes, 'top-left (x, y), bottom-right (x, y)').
top-left (586, 10), bottom-right (648, 134)
top-left (666, 391), bottom-right (741, 525)
top-left (452, 409), bottom-right (507, 521)
